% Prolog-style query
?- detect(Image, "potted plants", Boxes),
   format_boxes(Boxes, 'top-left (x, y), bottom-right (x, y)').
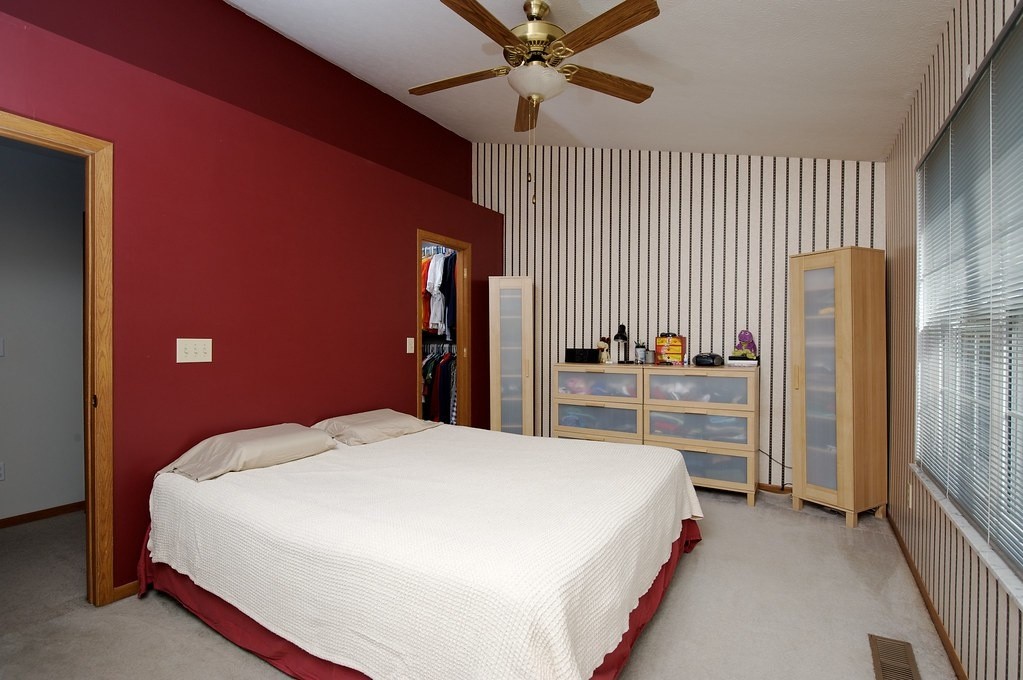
top-left (634, 341), bottom-right (646, 365)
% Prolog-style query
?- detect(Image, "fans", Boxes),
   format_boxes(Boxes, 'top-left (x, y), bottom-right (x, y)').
top-left (407, 0), bottom-right (661, 133)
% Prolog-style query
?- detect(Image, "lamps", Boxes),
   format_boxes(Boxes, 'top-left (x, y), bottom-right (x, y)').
top-left (614, 324), bottom-right (628, 365)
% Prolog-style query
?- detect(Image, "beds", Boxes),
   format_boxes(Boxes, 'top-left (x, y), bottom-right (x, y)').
top-left (135, 422), bottom-right (704, 680)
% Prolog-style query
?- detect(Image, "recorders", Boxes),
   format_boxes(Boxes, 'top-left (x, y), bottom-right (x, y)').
top-left (693, 352), bottom-right (723, 366)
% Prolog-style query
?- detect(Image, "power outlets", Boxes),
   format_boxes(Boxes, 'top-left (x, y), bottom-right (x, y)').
top-left (177, 338), bottom-right (213, 363)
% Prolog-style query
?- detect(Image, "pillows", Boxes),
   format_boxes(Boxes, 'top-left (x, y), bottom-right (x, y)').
top-left (311, 408), bottom-right (443, 447)
top-left (156, 422), bottom-right (337, 482)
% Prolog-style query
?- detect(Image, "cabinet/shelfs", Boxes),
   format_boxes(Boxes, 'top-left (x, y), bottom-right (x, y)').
top-left (552, 364), bottom-right (760, 508)
top-left (788, 246), bottom-right (889, 528)
top-left (489, 275), bottom-right (536, 436)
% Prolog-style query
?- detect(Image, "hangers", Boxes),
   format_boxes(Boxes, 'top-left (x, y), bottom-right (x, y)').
top-left (422, 344), bottom-right (455, 353)
top-left (421, 245), bottom-right (454, 256)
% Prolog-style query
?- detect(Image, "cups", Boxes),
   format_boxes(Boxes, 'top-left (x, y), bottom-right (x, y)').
top-left (646, 351), bottom-right (655, 363)
top-left (635, 348), bottom-right (646, 364)
